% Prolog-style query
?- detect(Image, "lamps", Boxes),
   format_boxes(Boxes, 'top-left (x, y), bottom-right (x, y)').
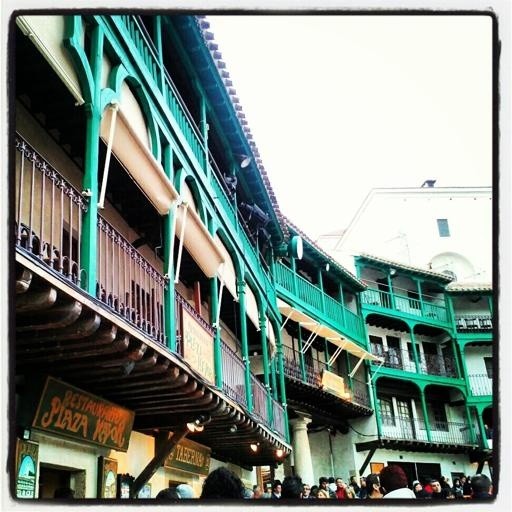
top-left (185, 415), bottom-right (208, 434)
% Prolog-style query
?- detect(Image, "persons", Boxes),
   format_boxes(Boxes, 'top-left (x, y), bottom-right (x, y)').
top-left (156, 463), bottom-right (495, 502)
